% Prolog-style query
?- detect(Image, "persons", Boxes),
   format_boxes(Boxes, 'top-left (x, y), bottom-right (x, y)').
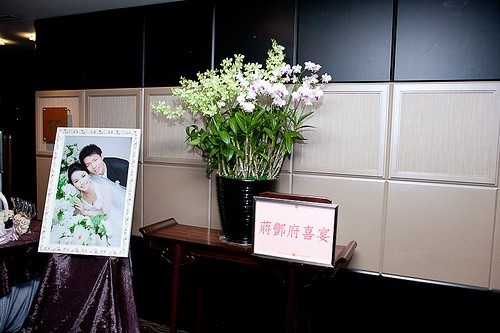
top-left (67, 144), bottom-right (129, 247)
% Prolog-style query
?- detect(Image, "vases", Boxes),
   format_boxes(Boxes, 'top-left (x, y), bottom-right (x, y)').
top-left (215, 173), bottom-right (279, 244)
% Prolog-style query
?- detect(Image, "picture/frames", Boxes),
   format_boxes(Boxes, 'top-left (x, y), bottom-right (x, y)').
top-left (36, 126), bottom-right (141, 258)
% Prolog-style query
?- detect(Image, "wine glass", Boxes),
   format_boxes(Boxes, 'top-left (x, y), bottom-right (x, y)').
top-left (10, 196), bottom-right (36, 235)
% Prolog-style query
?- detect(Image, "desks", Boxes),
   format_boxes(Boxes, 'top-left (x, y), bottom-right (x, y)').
top-left (139, 218), bottom-right (357, 333)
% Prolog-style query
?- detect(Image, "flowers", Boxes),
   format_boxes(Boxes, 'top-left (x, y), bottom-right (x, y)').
top-left (150, 38), bottom-right (332, 179)
